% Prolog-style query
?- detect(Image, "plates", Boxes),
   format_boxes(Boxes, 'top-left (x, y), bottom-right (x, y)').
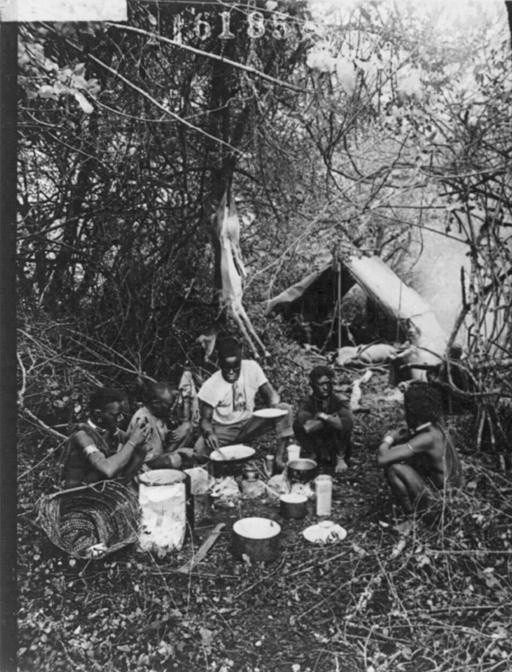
top-left (252, 408), bottom-right (289, 422)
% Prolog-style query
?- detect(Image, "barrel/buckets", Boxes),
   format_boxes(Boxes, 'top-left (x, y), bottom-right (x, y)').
top-left (138, 469), bottom-right (187, 554)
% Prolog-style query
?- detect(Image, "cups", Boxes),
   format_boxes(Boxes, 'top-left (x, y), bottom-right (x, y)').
top-left (315, 473), bottom-right (333, 517)
top-left (286, 444), bottom-right (300, 460)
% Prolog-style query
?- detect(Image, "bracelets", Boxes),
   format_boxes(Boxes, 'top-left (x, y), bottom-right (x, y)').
top-left (382, 436), bottom-right (394, 446)
top-left (128, 441), bottom-right (136, 448)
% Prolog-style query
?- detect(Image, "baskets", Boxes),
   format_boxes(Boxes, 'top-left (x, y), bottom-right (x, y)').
top-left (36, 481), bottom-right (142, 560)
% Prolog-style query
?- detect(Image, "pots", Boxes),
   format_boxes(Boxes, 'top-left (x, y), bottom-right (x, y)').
top-left (207, 443), bottom-right (258, 465)
top-left (286, 459), bottom-right (319, 479)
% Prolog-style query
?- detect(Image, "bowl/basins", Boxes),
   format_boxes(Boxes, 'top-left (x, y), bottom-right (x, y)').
top-left (232, 515), bottom-right (281, 563)
top-left (280, 494), bottom-right (308, 519)
top-left (303, 522), bottom-right (347, 545)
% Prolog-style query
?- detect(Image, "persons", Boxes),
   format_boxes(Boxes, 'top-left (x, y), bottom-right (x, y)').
top-left (120, 382), bottom-right (193, 484)
top-left (427, 344), bottom-right (465, 401)
top-left (62, 388), bottom-right (153, 492)
top-left (377, 382), bottom-right (461, 514)
top-left (194, 337), bottom-right (293, 474)
top-left (293, 365), bottom-right (353, 474)
